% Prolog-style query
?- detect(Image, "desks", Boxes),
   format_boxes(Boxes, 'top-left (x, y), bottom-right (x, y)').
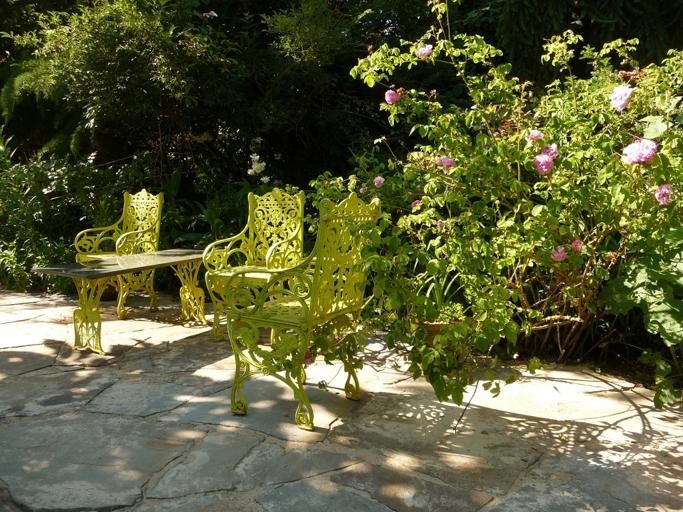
top-left (27, 246), bottom-right (209, 351)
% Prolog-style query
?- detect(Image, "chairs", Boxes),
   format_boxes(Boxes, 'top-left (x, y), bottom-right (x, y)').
top-left (221, 191), bottom-right (381, 433)
top-left (201, 188), bottom-right (307, 348)
top-left (74, 189), bottom-right (164, 319)
